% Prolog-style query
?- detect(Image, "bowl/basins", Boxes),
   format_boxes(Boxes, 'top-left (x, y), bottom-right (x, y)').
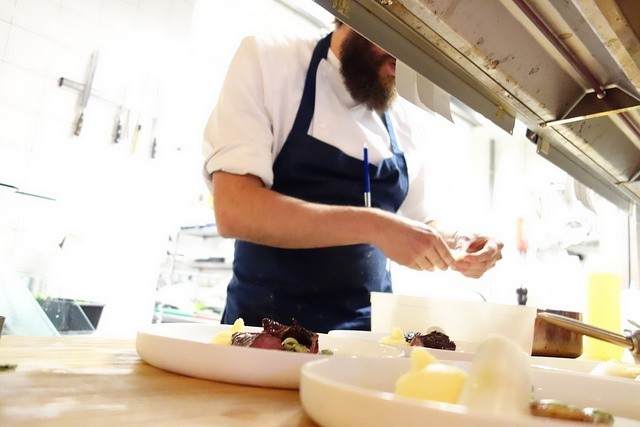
top-left (482, 299), bottom-right (537, 357)
top-left (370, 291), bottom-right (479, 351)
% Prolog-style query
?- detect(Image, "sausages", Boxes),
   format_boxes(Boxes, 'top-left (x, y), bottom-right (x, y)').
top-left (531, 400), bottom-right (613, 423)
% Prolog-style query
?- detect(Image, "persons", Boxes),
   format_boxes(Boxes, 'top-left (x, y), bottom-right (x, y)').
top-left (200, 16), bottom-right (505, 333)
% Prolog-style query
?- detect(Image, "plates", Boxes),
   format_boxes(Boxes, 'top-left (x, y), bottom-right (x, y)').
top-left (136, 322), bottom-right (405, 390)
top-left (298, 358), bottom-right (640, 427)
